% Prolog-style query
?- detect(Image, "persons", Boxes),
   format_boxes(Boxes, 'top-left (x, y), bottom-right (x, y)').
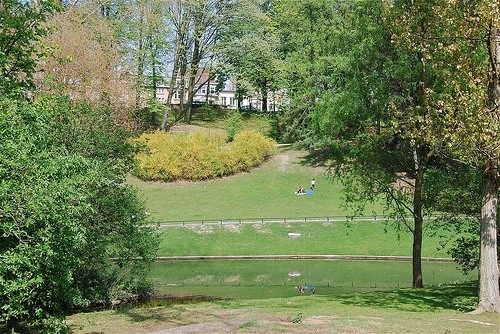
top-left (296, 178), bottom-right (317, 196)
